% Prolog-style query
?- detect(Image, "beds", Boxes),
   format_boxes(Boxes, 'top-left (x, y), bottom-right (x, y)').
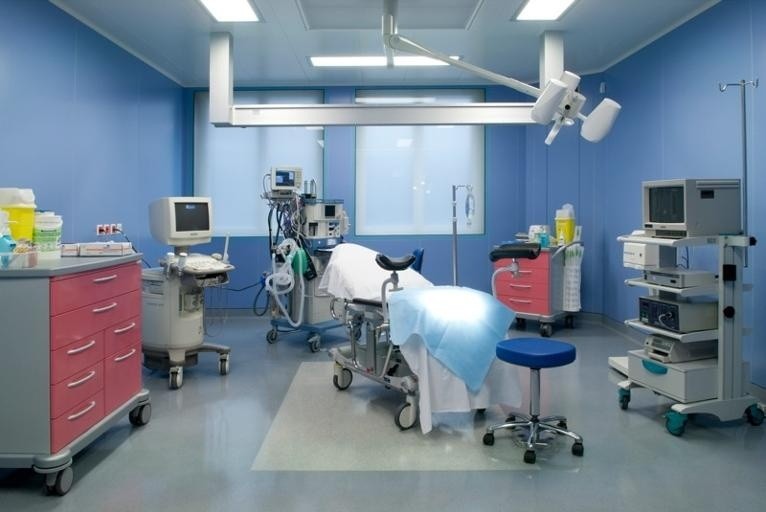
top-left (319, 243), bottom-right (520, 430)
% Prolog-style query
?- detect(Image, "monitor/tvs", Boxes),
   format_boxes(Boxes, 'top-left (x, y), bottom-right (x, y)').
top-left (270, 166), bottom-right (302, 190)
top-left (149, 197), bottom-right (212, 247)
top-left (641, 178), bottom-right (742, 237)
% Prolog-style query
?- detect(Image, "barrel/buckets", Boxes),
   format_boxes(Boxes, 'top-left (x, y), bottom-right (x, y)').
top-left (33, 215), bottom-right (64, 258)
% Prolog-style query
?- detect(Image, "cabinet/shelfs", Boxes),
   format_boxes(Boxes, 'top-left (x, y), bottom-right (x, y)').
top-left (0, 253), bottom-right (150, 495)
top-left (494, 247), bottom-right (566, 322)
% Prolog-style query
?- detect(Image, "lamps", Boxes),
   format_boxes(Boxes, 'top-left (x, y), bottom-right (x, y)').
top-left (529, 70), bottom-right (623, 146)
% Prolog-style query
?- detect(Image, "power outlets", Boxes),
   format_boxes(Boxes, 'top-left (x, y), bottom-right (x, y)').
top-left (97, 222), bottom-right (121, 236)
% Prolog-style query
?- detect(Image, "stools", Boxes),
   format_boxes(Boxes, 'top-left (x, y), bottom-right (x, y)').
top-left (482, 337), bottom-right (584, 464)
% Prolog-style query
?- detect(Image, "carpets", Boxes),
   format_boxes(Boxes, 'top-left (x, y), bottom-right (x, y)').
top-left (246, 363), bottom-right (538, 472)
